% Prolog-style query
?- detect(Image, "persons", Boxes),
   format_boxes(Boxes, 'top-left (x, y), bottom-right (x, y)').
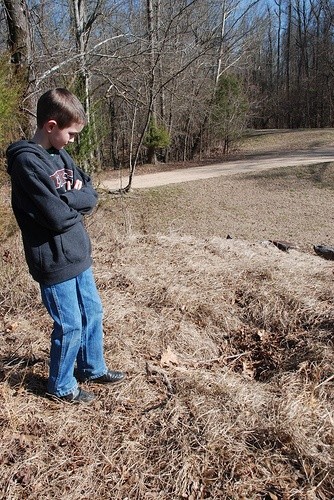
top-left (5, 88), bottom-right (128, 403)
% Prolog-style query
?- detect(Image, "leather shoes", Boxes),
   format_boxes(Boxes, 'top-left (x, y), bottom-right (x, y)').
top-left (76, 368), bottom-right (128, 385)
top-left (45, 387), bottom-right (97, 403)
